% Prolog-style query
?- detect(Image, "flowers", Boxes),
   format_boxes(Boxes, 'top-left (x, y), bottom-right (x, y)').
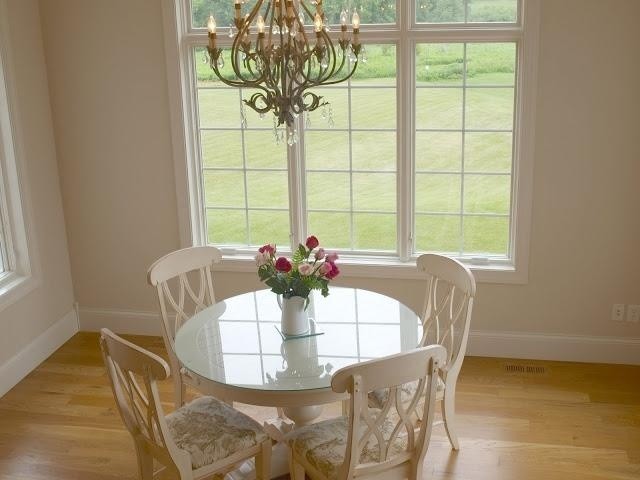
top-left (253, 236), bottom-right (342, 302)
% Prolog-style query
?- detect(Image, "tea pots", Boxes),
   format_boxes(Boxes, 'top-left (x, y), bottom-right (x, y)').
top-left (277, 293), bottom-right (309, 335)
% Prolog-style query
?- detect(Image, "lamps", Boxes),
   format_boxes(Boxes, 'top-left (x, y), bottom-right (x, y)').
top-left (201, 0), bottom-right (362, 147)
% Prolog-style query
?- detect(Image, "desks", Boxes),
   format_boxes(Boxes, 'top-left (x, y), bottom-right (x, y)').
top-left (173, 285), bottom-right (426, 480)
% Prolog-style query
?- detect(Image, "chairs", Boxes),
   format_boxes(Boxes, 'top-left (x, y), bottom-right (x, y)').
top-left (146, 245), bottom-right (281, 417)
top-left (341, 251), bottom-right (479, 452)
top-left (98, 328), bottom-right (273, 480)
top-left (283, 343), bottom-right (448, 480)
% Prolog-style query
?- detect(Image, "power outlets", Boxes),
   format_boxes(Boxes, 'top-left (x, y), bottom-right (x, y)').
top-left (612, 302), bottom-right (639, 325)
